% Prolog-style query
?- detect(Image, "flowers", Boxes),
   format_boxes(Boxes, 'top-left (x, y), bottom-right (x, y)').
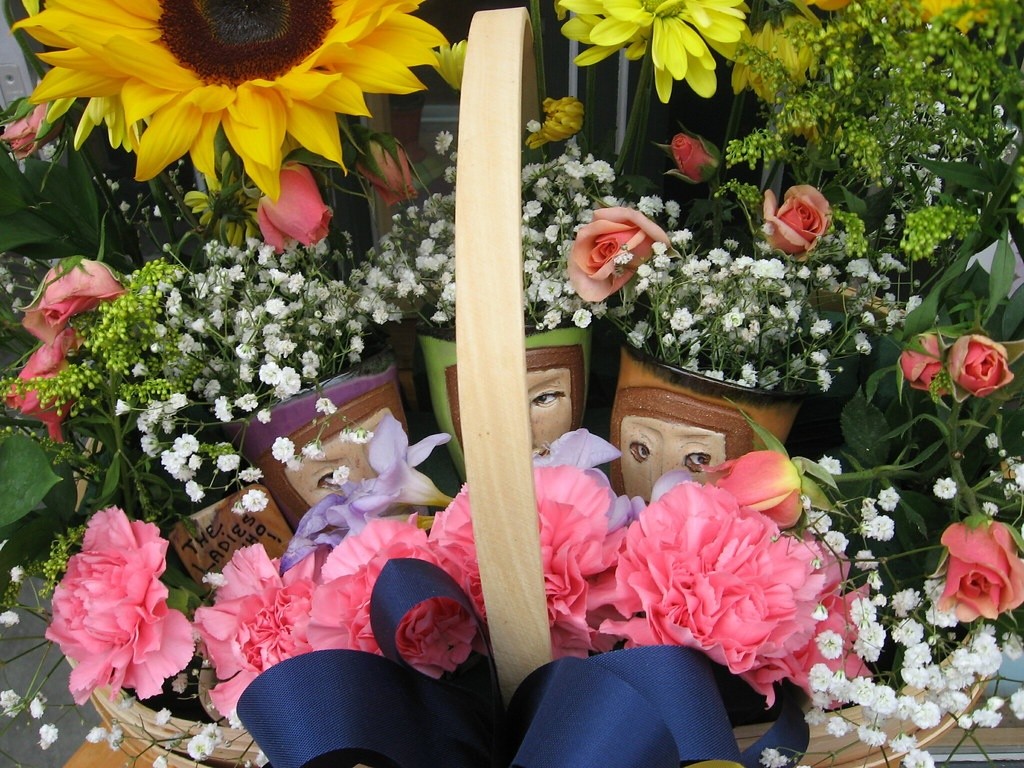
top-left (0, 0), bottom-right (1024, 767)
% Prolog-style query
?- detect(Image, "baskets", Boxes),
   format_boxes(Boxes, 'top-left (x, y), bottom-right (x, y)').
top-left (85, 7), bottom-right (990, 768)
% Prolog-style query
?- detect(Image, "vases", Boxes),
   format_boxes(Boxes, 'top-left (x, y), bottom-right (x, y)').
top-left (415, 314), bottom-right (591, 481)
top-left (167, 484), bottom-right (291, 595)
top-left (221, 349), bottom-right (410, 529)
top-left (607, 339), bottom-right (814, 506)
